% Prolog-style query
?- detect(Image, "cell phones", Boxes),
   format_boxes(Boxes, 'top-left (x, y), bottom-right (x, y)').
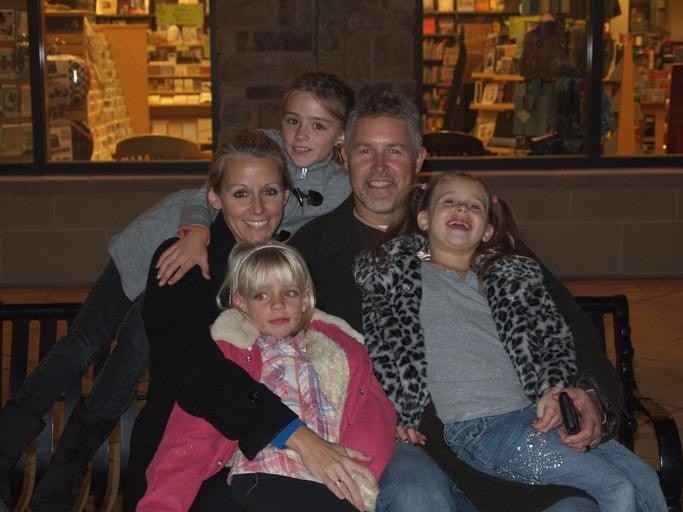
top-left (559, 390), bottom-right (580, 435)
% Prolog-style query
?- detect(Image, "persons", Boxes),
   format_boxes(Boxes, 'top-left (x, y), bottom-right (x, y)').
top-left (277, 85), bottom-right (631, 512)
top-left (351, 165), bottom-right (669, 511)
top-left (121, 129), bottom-right (380, 512)
top-left (134, 235), bottom-right (399, 512)
top-left (0, 68), bottom-right (354, 512)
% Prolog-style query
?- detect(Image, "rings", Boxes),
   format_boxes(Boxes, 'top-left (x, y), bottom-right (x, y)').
top-left (335, 479), bottom-right (342, 485)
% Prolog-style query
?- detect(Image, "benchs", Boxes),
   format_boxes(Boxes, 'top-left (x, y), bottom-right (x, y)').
top-left (0, 295), bottom-right (683, 512)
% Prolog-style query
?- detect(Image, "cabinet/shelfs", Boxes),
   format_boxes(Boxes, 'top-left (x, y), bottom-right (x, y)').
top-left (0, 0), bottom-right (212, 163)
top-left (424, 1), bottom-right (683, 157)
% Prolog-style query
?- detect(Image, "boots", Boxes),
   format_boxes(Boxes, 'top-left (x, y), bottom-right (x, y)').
top-left (1, 399), bottom-right (45, 512)
top-left (33, 459), bottom-right (87, 511)
